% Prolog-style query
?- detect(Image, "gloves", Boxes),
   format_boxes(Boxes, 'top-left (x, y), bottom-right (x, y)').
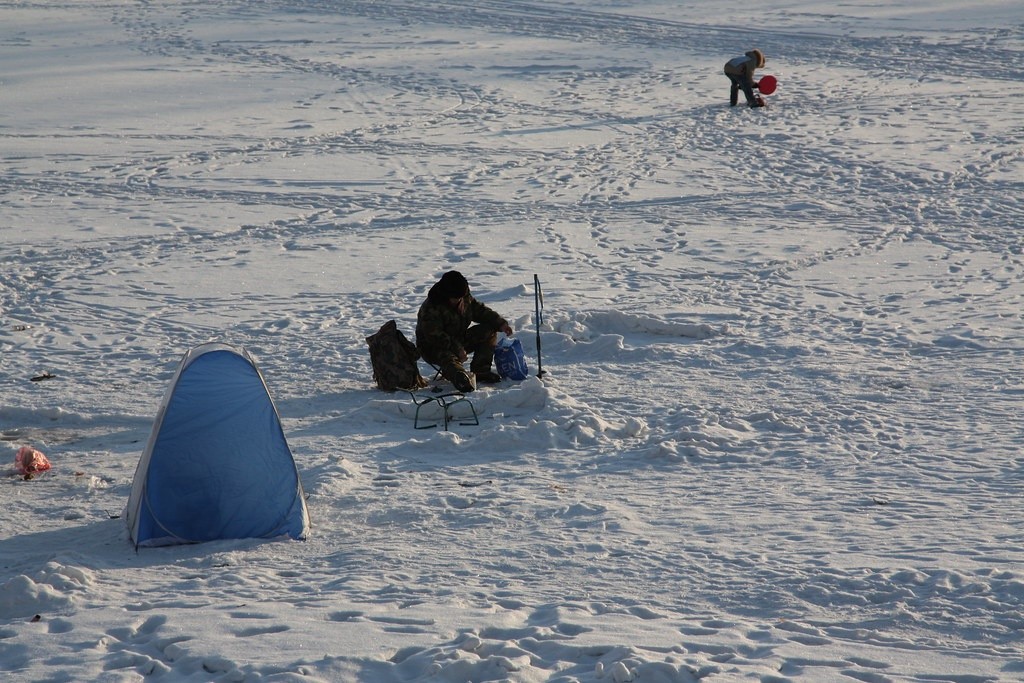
top-left (752, 83), bottom-right (760, 89)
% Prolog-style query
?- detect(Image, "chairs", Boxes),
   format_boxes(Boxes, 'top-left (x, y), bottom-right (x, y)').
top-left (394, 386), bottom-right (479, 432)
top-left (425, 362), bottom-right (444, 381)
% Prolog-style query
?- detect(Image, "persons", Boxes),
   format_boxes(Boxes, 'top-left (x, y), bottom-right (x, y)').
top-left (724, 48), bottom-right (766, 110)
top-left (415, 270), bottom-right (513, 394)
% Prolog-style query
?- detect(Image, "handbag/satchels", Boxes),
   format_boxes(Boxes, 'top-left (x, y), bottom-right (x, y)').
top-left (494, 335), bottom-right (528, 381)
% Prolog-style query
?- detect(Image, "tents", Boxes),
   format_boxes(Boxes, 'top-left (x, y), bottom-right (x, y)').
top-left (124, 341), bottom-right (313, 555)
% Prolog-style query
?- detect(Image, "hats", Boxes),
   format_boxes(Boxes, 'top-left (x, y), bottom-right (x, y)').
top-left (753, 49), bottom-right (766, 68)
top-left (436, 271), bottom-right (468, 299)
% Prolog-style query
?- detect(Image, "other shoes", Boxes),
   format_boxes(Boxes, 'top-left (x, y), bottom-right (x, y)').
top-left (452, 370), bottom-right (475, 393)
top-left (749, 100), bottom-right (762, 109)
top-left (470, 368), bottom-right (502, 384)
top-left (730, 100), bottom-right (737, 106)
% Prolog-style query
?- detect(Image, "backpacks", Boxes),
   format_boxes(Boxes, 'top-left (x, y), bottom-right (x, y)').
top-left (365, 318), bottom-right (428, 392)
